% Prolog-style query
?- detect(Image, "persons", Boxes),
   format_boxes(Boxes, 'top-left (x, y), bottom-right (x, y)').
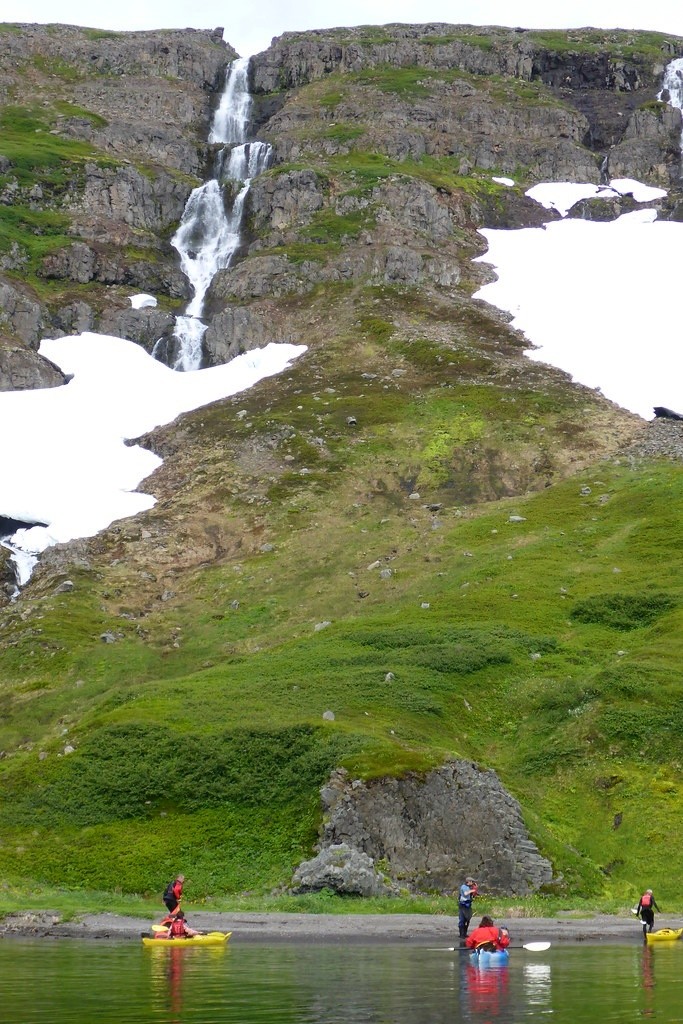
top-left (168, 912), bottom-right (199, 938)
top-left (466, 915), bottom-right (510, 950)
top-left (458, 877), bottom-right (476, 939)
top-left (636, 889), bottom-right (661, 940)
top-left (164, 874), bottom-right (185, 914)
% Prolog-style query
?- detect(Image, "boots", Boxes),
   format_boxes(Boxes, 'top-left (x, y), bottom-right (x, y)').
top-left (459, 925), bottom-right (469, 939)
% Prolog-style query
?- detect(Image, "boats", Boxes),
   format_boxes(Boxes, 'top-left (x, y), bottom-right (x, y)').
top-left (470, 950), bottom-right (510, 962)
top-left (142, 932), bottom-right (232, 945)
top-left (646, 929), bottom-right (683, 941)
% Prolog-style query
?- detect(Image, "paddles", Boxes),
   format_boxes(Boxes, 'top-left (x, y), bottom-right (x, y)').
top-left (630, 908), bottom-right (645, 924)
top-left (151, 922), bottom-right (225, 936)
top-left (427, 941), bottom-right (551, 953)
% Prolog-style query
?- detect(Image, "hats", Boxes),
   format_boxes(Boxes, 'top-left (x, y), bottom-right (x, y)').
top-left (466, 877), bottom-right (475, 881)
